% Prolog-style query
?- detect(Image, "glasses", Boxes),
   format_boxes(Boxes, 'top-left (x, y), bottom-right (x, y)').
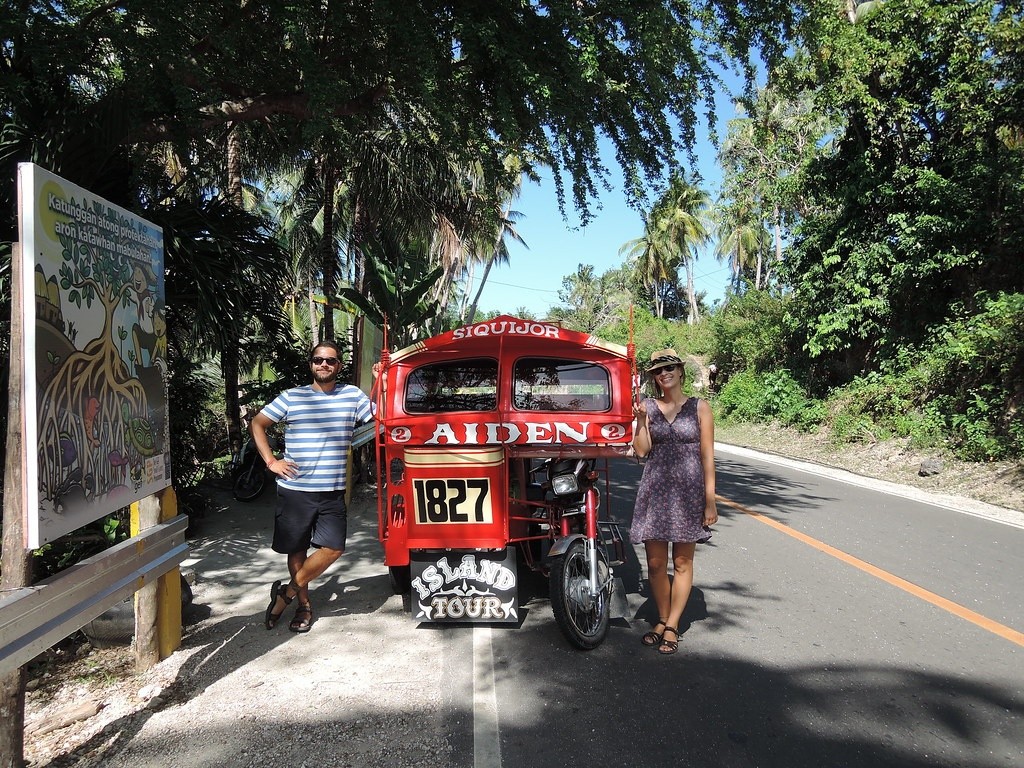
top-left (310, 356), bottom-right (340, 366)
top-left (652, 364), bottom-right (680, 375)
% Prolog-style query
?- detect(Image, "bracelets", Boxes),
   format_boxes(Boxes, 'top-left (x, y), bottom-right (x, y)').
top-left (267, 457), bottom-right (277, 468)
top-left (383, 389), bottom-right (386, 392)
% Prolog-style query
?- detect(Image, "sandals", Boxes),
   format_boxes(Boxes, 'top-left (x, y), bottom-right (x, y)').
top-left (642, 621), bottom-right (667, 645)
top-left (266, 580), bottom-right (294, 629)
top-left (289, 604), bottom-right (312, 632)
top-left (659, 626), bottom-right (684, 653)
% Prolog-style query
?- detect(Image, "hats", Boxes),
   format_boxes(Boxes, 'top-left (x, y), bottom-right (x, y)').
top-left (646, 348), bottom-right (686, 375)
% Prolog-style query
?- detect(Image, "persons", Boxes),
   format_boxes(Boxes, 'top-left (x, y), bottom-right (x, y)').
top-left (252, 342), bottom-right (388, 633)
top-left (630, 347), bottom-right (720, 654)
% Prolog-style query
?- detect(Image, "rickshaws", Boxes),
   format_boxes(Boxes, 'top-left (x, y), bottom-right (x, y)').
top-left (369, 314), bottom-right (640, 652)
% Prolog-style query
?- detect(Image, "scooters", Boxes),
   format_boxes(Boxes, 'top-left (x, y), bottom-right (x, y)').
top-left (230, 413), bottom-right (285, 503)
